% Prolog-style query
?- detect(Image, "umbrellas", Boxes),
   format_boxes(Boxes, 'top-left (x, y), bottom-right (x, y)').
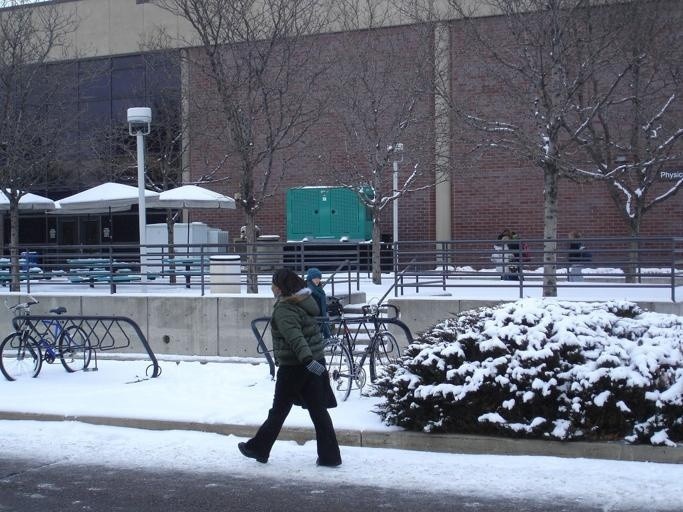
top-left (45, 181), bottom-right (160, 294)
top-left (156, 185), bottom-right (236, 288)
top-left (0, 187), bottom-right (56, 259)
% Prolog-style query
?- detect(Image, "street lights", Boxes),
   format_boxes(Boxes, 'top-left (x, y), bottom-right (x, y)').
top-left (385, 141), bottom-right (405, 272)
top-left (124, 106), bottom-right (152, 283)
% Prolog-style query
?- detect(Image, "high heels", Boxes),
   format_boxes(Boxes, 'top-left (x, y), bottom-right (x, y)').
top-left (238, 440), bottom-right (268, 463)
top-left (316, 454), bottom-right (342, 467)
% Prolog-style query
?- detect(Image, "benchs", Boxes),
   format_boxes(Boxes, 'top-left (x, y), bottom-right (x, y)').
top-left (0, 255), bottom-right (209, 290)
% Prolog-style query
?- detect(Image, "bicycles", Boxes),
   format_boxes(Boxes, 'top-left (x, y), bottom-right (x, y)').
top-left (0, 291), bottom-right (90, 384)
top-left (322, 302), bottom-right (400, 401)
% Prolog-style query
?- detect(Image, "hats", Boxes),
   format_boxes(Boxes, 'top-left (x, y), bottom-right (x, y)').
top-left (305, 267), bottom-right (323, 281)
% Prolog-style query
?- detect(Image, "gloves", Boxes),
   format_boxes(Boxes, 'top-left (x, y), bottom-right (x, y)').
top-left (303, 359), bottom-right (329, 380)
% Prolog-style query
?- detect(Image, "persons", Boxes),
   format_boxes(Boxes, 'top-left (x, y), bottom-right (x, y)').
top-left (304, 267), bottom-right (331, 339)
top-left (238, 267), bottom-right (343, 466)
top-left (567, 231), bottom-right (592, 268)
